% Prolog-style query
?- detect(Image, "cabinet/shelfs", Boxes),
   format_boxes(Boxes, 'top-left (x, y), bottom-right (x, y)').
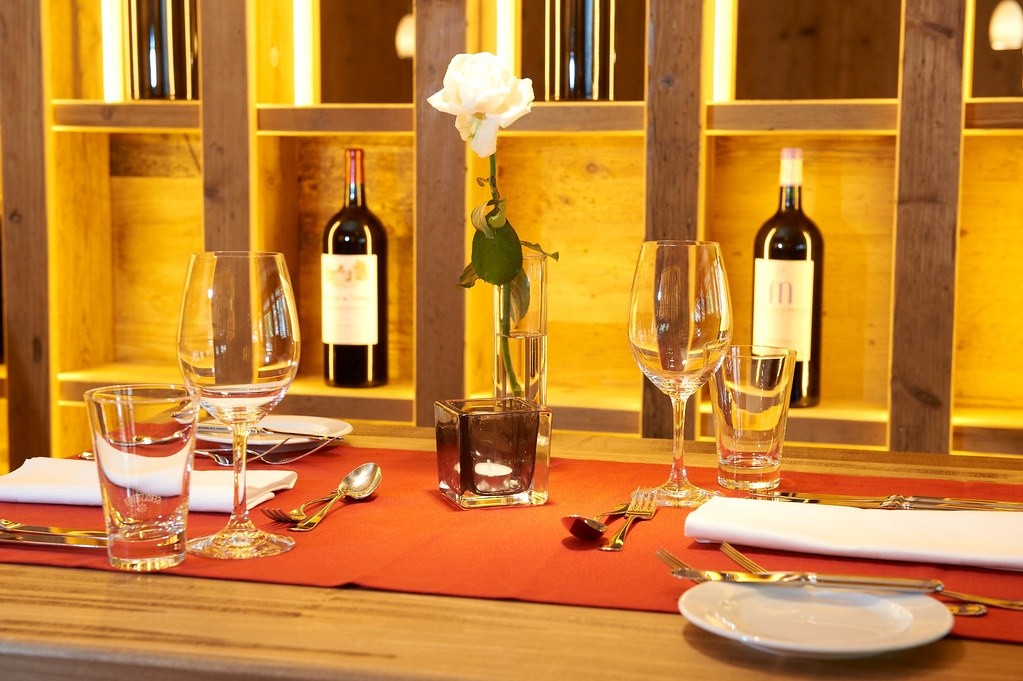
top-left (38, 1), bottom-right (1020, 454)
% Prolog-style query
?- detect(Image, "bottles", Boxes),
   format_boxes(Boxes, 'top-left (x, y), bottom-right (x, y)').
top-left (320, 149), bottom-right (388, 388)
top-left (751, 148), bottom-right (825, 408)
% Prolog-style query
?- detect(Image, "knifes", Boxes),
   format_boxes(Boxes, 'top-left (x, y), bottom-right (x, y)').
top-left (669, 567), bottom-right (944, 595)
top-left (196, 425), bottom-right (345, 441)
top-left (1, 518), bottom-right (108, 549)
top-left (745, 490), bottom-right (1022, 513)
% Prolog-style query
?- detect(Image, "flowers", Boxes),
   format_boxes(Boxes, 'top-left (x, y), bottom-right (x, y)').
top-left (427, 51), bottom-right (560, 403)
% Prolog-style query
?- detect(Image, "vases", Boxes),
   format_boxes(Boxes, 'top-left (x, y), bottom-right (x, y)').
top-left (431, 251), bottom-right (546, 507)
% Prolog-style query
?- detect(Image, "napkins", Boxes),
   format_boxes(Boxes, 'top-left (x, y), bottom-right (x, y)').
top-left (0, 457), bottom-right (299, 516)
top-left (684, 498), bottom-right (1023, 576)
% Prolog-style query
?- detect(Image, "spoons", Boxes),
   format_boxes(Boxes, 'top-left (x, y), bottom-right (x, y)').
top-left (561, 504), bottom-right (629, 542)
top-left (286, 462), bottom-right (383, 532)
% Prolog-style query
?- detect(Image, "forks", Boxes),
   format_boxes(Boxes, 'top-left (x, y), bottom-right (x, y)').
top-left (260, 492), bottom-right (338, 522)
top-left (656, 541), bottom-right (1023, 616)
top-left (78, 435), bottom-right (338, 466)
top-left (597, 486), bottom-right (658, 551)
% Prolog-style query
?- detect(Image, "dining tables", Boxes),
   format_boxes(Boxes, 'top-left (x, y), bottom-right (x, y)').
top-left (1, 416), bottom-right (1023, 680)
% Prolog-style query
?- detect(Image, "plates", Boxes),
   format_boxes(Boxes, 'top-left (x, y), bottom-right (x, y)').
top-left (677, 581), bottom-right (954, 659)
top-left (196, 415), bottom-right (353, 453)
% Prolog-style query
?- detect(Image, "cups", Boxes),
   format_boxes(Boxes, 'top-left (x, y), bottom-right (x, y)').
top-left (434, 395), bottom-right (552, 512)
top-left (709, 346), bottom-right (797, 491)
top-left (83, 383), bottom-right (201, 570)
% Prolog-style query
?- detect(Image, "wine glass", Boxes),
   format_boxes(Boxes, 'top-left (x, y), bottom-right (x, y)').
top-left (177, 252), bottom-right (301, 560)
top-left (627, 240), bottom-right (733, 506)
